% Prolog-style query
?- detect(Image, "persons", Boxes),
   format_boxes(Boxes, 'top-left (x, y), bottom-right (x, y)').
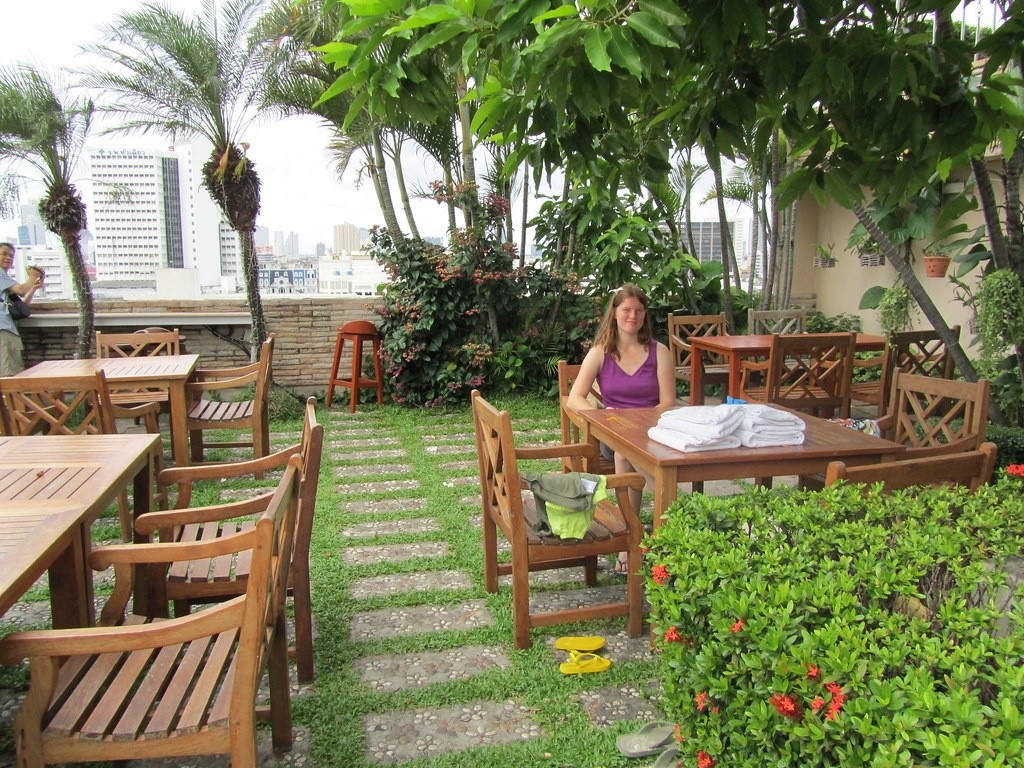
top-left (566, 284), bottom-right (677, 574)
top-left (0, 242), bottom-right (45, 376)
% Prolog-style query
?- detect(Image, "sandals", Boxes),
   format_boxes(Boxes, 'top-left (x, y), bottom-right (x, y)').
top-left (560, 653), bottom-right (614, 675)
top-left (555, 636), bottom-right (606, 655)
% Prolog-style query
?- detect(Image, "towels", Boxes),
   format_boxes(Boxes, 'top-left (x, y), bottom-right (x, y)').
top-left (647, 403), bottom-right (807, 453)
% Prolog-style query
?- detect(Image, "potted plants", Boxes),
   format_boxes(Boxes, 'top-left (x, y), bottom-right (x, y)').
top-left (921, 242), bottom-right (952, 278)
top-left (812, 241), bottom-right (837, 267)
top-left (859, 236), bottom-right (888, 266)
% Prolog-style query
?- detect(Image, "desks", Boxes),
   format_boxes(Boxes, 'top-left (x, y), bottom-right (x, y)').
top-left (7, 352), bottom-right (201, 476)
top-left (576, 401), bottom-right (904, 640)
top-left (1, 433), bottom-right (164, 668)
top-left (686, 333), bottom-right (890, 408)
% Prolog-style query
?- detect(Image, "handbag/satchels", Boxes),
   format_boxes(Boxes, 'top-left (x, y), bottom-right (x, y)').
top-left (0, 292), bottom-right (30, 321)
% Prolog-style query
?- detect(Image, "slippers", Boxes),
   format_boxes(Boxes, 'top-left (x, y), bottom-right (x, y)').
top-left (615, 552), bottom-right (628, 575)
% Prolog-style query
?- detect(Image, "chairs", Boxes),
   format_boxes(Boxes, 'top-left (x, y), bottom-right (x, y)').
top-left (472, 306), bottom-right (997, 648)
top-left (1, 327), bottom-right (328, 767)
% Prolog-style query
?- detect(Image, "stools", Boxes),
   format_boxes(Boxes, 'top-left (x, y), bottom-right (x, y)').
top-left (327, 331), bottom-right (383, 415)
